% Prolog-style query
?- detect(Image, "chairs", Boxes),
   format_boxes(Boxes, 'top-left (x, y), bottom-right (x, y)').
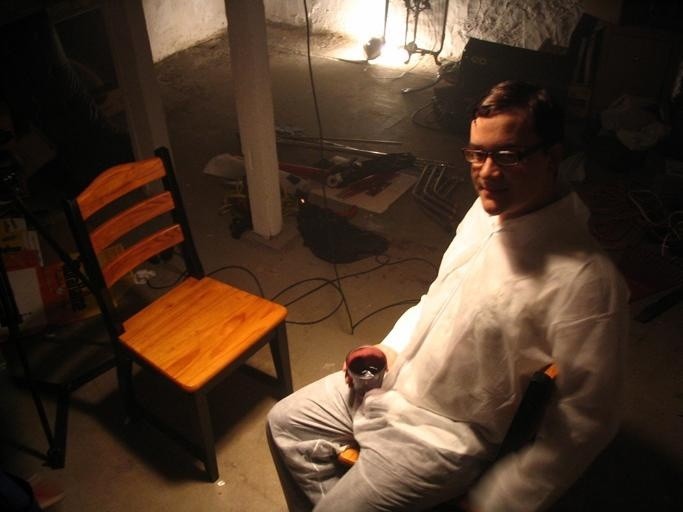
top-left (334, 362), bottom-right (561, 512)
top-left (62, 147), bottom-right (303, 485)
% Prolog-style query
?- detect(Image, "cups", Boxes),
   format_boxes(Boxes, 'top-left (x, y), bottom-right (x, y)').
top-left (345, 346), bottom-right (387, 396)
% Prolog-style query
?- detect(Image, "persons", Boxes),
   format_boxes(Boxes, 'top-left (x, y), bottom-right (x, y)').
top-left (265, 80), bottom-right (631, 512)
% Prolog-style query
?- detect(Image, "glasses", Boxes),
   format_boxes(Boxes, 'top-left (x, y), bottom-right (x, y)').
top-left (460, 141), bottom-right (554, 167)
